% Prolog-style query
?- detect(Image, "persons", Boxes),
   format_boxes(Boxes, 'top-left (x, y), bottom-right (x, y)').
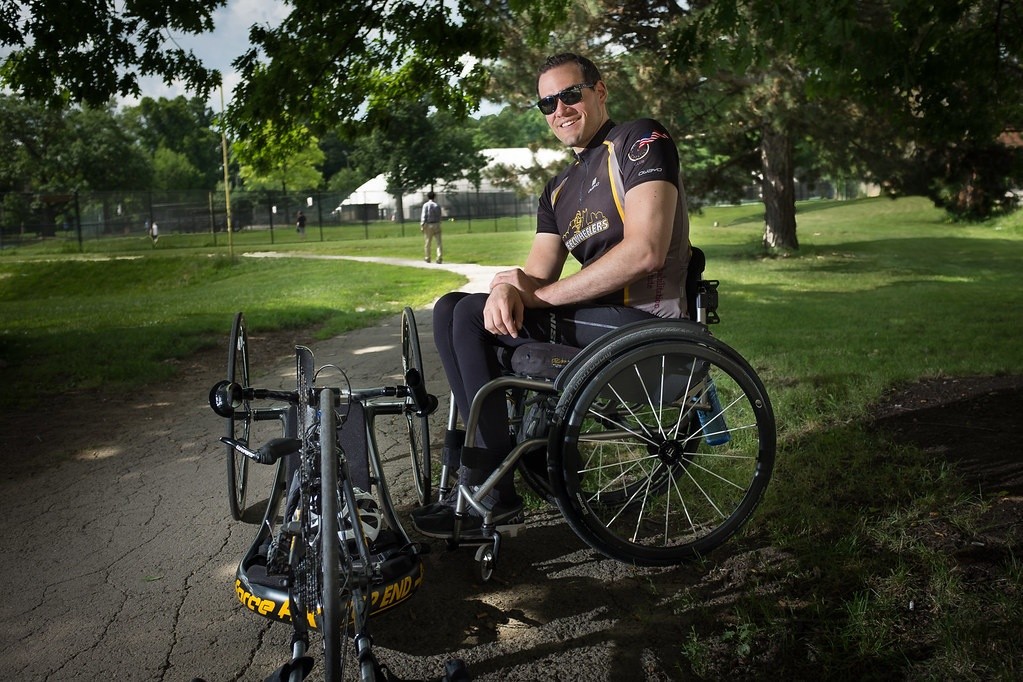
top-left (150, 221), bottom-right (159, 249)
top-left (420, 192), bottom-right (442, 264)
top-left (410, 52), bottom-right (712, 541)
top-left (296, 211), bottom-right (306, 236)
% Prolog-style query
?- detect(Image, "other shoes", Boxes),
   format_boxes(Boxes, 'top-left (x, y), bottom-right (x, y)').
top-left (424, 257), bottom-right (430, 263)
top-left (436, 259), bottom-right (441, 264)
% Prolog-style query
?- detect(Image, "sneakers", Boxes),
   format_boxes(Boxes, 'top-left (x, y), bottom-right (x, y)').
top-left (411, 495), bottom-right (527, 539)
top-left (410, 482), bottom-right (458, 520)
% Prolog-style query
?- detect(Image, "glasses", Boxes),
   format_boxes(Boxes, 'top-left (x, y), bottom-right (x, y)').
top-left (537, 83), bottom-right (594, 115)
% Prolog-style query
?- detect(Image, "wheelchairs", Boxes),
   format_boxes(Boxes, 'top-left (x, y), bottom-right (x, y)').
top-left (438, 247), bottom-right (777, 585)
top-left (212, 307), bottom-right (437, 682)
